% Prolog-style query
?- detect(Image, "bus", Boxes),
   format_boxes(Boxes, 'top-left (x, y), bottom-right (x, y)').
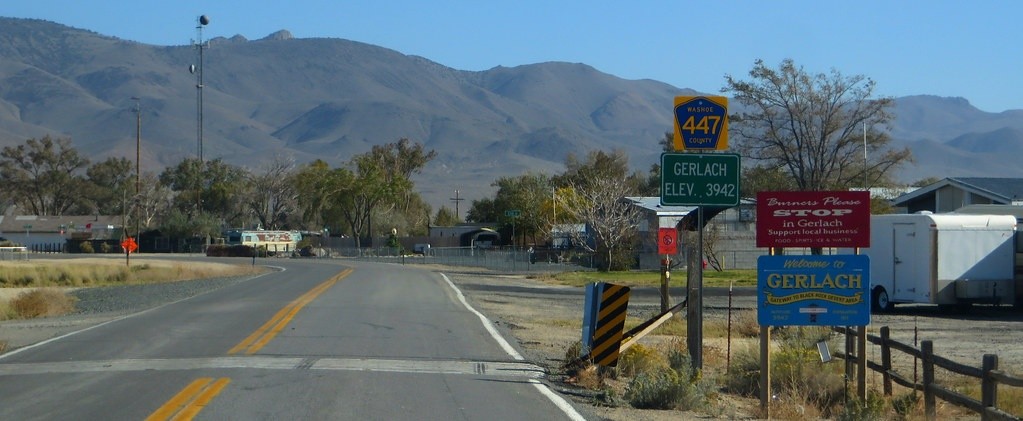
top-left (239, 229), bottom-right (303, 254)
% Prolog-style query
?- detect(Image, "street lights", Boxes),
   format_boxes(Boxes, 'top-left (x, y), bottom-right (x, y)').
top-left (131, 95), bottom-right (142, 254)
top-left (188, 14), bottom-right (210, 256)
